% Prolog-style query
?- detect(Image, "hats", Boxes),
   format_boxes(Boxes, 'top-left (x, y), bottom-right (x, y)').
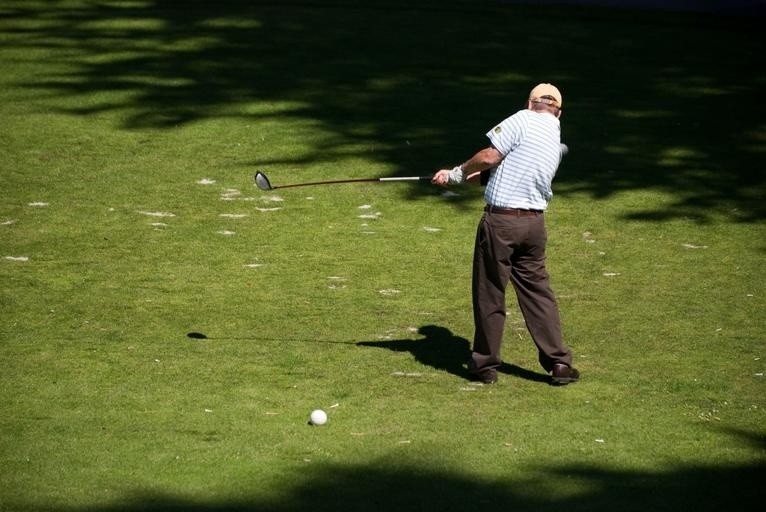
top-left (529, 83), bottom-right (562, 109)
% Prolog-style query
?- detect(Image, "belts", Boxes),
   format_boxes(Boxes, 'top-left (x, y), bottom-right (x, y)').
top-left (484, 203), bottom-right (542, 216)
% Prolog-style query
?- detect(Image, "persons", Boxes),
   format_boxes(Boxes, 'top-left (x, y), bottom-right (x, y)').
top-left (428, 82), bottom-right (581, 386)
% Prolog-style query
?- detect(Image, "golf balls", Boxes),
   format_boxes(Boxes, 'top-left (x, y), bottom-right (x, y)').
top-left (310, 410), bottom-right (327, 423)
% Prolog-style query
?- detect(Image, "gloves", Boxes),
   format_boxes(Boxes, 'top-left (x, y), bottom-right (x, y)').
top-left (447, 166), bottom-right (466, 186)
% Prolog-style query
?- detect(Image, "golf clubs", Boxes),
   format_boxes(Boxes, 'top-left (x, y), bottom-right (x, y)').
top-left (255, 170), bottom-right (469, 190)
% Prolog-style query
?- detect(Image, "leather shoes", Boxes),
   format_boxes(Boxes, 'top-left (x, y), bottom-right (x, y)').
top-left (551, 364), bottom-right (579, 384)
top-left (474, 369), bottom-right (498, 384)
